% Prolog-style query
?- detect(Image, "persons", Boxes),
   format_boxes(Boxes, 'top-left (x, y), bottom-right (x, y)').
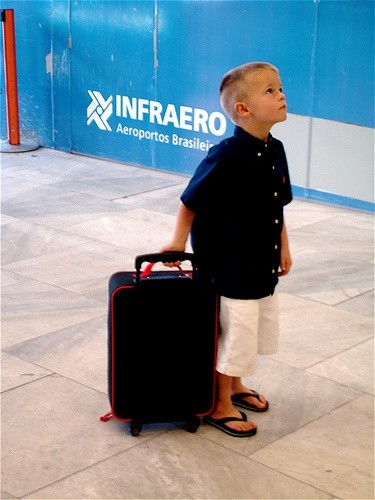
top-left (161, 61), bottom-right (293, 437)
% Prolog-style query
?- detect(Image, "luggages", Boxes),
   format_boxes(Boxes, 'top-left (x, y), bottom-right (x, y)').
top-left (99, 253), bottom-right (218, 436)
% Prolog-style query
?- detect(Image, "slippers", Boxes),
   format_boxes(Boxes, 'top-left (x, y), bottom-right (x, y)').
top-left (230, 390), bottom-right (268, 413)
top-left (203, 410), bottom-right (257, 437)
